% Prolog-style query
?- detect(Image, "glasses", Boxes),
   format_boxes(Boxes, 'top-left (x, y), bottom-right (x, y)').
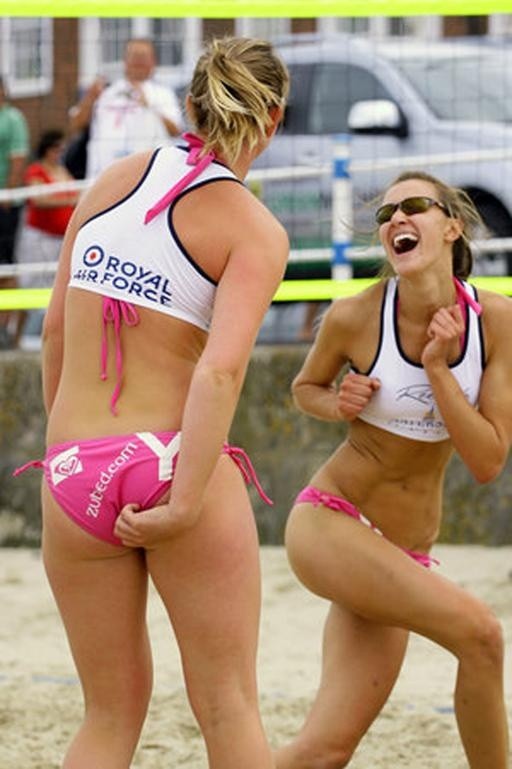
top-left (374, 194), bottom-right (459, 226)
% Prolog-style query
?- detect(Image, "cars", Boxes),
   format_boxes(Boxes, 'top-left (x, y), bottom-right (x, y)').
top-left (164, 32), bottom-right (512, 283)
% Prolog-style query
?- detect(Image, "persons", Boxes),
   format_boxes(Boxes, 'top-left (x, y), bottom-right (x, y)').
top-left (34, 35), bottom-right (293, 768)
top-left (0, 74), bottom-right (32, 350)
top-left (276, 168), bottom-right (512, 768)
top-left (67, 36), bottom-right (188, 180)
top-left (10, 128), bottom-right (82, 344)
